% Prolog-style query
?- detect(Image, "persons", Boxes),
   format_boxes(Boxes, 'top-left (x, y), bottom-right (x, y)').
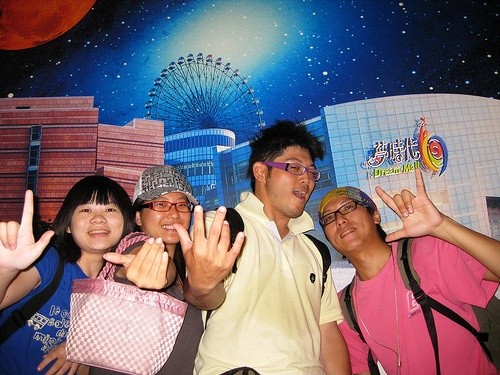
top-left (318, 169), bottom-right (500, 375)
top-left (1, 175), bottom-right (136, 375)
top-left (172, 119), bottom-right (352, 375)
top-left (89, 165), bottom-right (204, 375)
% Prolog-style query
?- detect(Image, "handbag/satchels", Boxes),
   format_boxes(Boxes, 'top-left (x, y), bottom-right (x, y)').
top-left (65, 233), bottom-right (188, 375)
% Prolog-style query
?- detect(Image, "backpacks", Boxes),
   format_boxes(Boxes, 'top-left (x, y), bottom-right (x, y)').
top-left (341, 237), bottom-right (500, 375)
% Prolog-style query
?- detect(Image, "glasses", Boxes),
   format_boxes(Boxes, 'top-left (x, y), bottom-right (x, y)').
top-left (143, 202), bottom-right (193, 212)
top-left (261, 162), bottom-right (320, 180)
top-left (320, 202), bottom-right (363, 226)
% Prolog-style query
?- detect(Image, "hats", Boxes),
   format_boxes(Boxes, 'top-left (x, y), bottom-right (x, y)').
top-left (132, 166), bottom-right (199, 204)
top-left (319, 187), bottom-right (377, 219)
